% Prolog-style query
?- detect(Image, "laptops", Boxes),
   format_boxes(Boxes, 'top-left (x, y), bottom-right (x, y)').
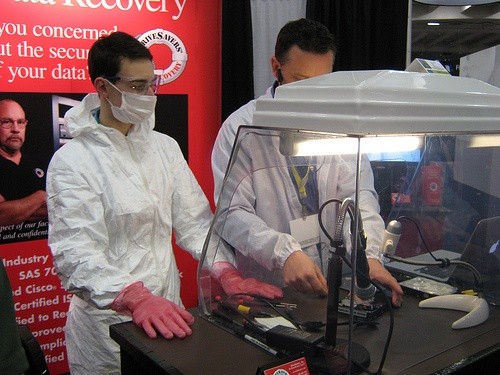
top-left (385, 216), bottom-right (500, 286)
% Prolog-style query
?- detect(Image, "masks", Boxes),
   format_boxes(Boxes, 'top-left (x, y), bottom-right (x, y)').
top-left (102, 78), bottom-right (157, 125)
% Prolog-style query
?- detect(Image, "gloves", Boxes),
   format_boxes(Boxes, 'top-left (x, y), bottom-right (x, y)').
top-left (110, 282), bottom-right (194, 342)
top-left (210, 262), bottom-right (282, 302)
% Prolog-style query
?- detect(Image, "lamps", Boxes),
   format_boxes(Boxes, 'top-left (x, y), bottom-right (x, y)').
top-left (279, 132), bottom-right (427, 156)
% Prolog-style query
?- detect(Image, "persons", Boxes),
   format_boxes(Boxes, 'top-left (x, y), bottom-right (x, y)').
top-left (212, 18), bottom-right (403, 306)
top-left (46, 31), bottom-right (284, 375)
top-left (0, 98), bottom-right (46, 226)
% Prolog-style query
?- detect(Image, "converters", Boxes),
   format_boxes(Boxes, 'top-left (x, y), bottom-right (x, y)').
top-left (264, 325), bottom-right (325, 356)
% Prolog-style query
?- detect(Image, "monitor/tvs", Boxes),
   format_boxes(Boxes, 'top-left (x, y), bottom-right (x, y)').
top-left (368, 146), bottom-right (423, 167)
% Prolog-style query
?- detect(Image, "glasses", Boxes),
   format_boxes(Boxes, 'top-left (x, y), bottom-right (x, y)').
top-left (1, 119), bottom-right (28, 128)
top-left (112, 76), bottom-right (159, 93)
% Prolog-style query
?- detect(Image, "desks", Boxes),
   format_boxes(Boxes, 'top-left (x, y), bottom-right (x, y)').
top-left (108, 271), bottom-right (500, 375)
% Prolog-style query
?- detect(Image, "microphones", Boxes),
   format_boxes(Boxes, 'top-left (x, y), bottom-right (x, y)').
top-left (380, 220), bottom-right (401, 256)
top-left (349, 220), bottom-right (376, 300)
